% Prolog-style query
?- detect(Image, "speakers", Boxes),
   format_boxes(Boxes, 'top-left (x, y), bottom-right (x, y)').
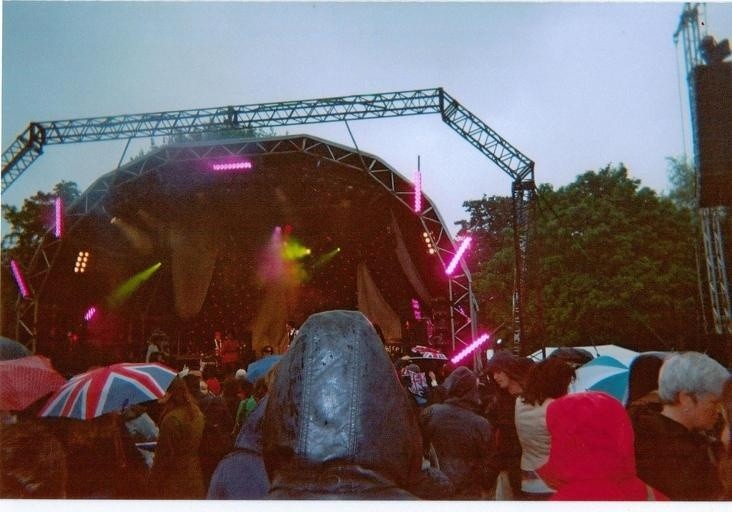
top-left (401, 320), bottom-right (429, 345)
top-left (224, 356), bottom-right (248, 370)
top-left (171, 354), bottom-right (200, 370)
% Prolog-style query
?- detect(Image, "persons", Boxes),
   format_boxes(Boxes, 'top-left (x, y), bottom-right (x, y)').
top-left (1, 308), bottom-right (731, 501)
